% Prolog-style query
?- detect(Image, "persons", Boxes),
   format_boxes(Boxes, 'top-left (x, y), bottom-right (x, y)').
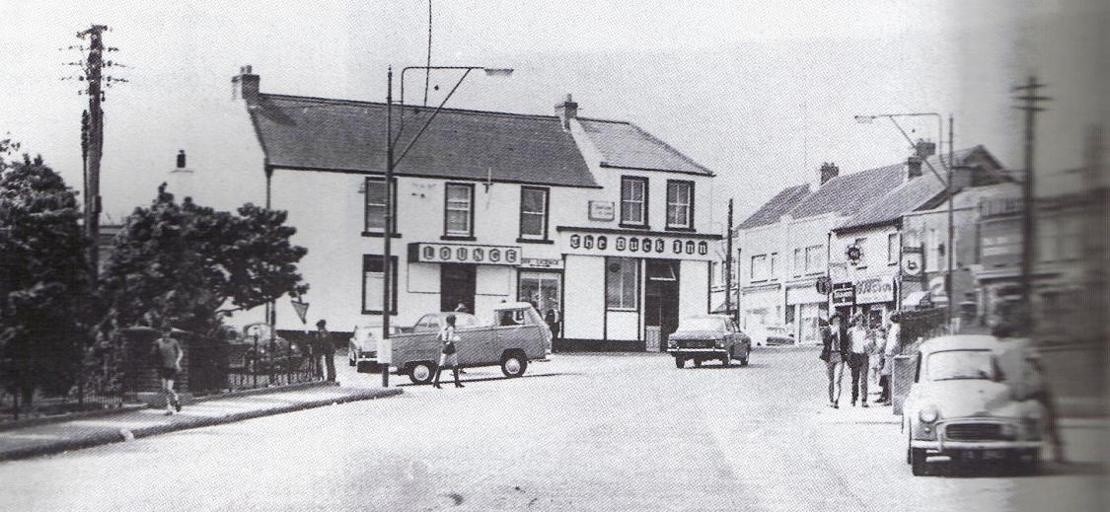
top-left (432, 312), bottom-right (465, 389)
top-left (500, 311), bottom-right (525, 325)
top-left (531, 300), bottom-right (544, 320)
top-left (819, 310), bottom-right (849, 410)
top-left (845, 310), bottom-right (872, 408)
top-left (314, 319), bottom-right (338, 381)
top-left (978, 319), bottom-right (1073, 468)
top-left (544, 302), bottom-right (562, 353)
top-left (454, 299), bottom-right (471, 313)
top-left (148, 320), bottom-right (184, 416)
top-left (867, 310), bottom-right (901, 407)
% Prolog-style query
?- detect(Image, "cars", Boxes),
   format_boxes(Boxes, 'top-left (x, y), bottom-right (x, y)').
top-left (900, 335), bottom-right (1055, 483)
top-left (766, 326), bottom-right (796, 345)
top-left (663, 313), bottom-right (756, 372)
top-left (348, 322), bottom-right (407, 374)
top-left (411, 312), bottom-right (482, 333)
top-left (221, 321), bottom-right (304, 374)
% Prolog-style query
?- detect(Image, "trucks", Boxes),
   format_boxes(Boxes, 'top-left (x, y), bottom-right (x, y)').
top-left (390, 300), bottom-right (553, 384)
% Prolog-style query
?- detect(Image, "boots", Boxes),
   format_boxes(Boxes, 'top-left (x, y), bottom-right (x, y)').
top-left (434, 365), bottom-right (445, 389)
top-left (453, 365), bottom-right (464, 387)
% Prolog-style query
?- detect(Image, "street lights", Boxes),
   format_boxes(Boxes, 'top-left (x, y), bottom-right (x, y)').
top-left (854, 112), bottom-right (960, 329)
top-left (383, 62), bottom-right (517, 389)
top-left (951, 162), bottom-right (1033, 335)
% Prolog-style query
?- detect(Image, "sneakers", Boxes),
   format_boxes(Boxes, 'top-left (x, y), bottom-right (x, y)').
top-left (164, 412), bottom-right (172, 417)
top-left (175, 401), bottom-right (180, 412)
top-left (834, 397), bottom-right (892, 407)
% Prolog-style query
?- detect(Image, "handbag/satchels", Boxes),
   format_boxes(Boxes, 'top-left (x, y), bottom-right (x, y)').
top-left (442, 342), bottom-right (456, 354)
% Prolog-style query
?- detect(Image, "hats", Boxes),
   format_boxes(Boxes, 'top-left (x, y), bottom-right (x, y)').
top-left (161, 322), bottom-right (171, 330)
top-left (829, 312), bottom-right (843, 325)
